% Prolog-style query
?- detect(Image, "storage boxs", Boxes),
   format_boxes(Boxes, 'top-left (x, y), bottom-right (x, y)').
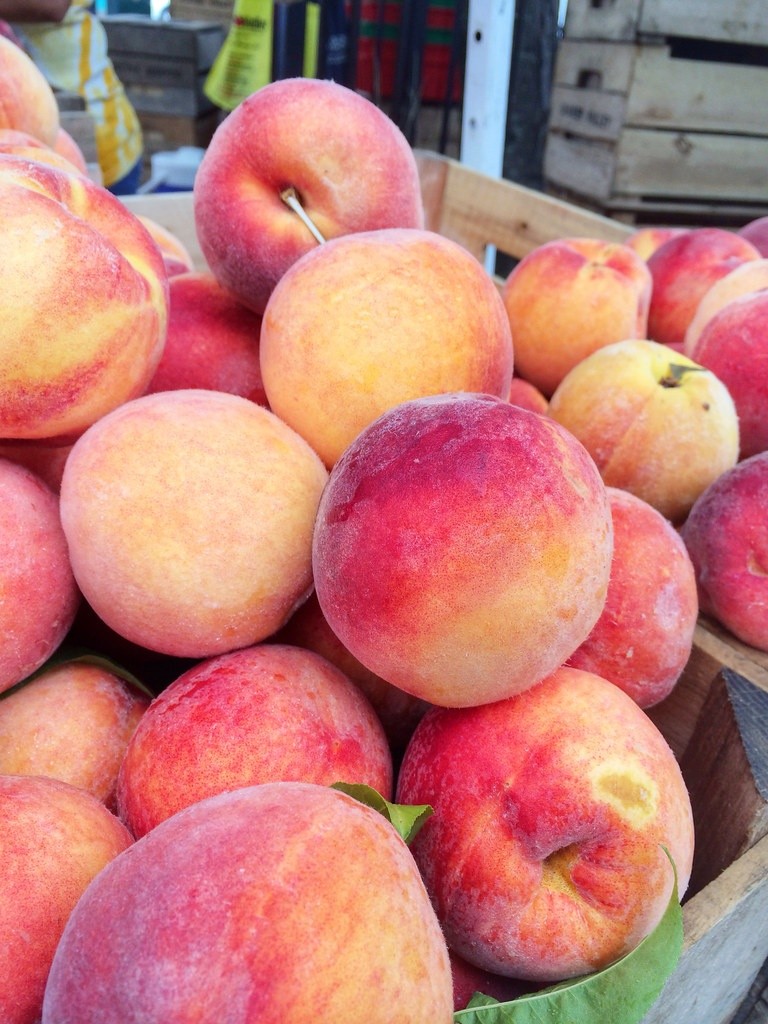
top-left (94, 0), bottom-right (767, 1023)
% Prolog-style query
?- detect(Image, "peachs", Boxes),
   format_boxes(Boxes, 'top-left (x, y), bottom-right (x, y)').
top-left (0, 22), bottom-right (768, 1024)
